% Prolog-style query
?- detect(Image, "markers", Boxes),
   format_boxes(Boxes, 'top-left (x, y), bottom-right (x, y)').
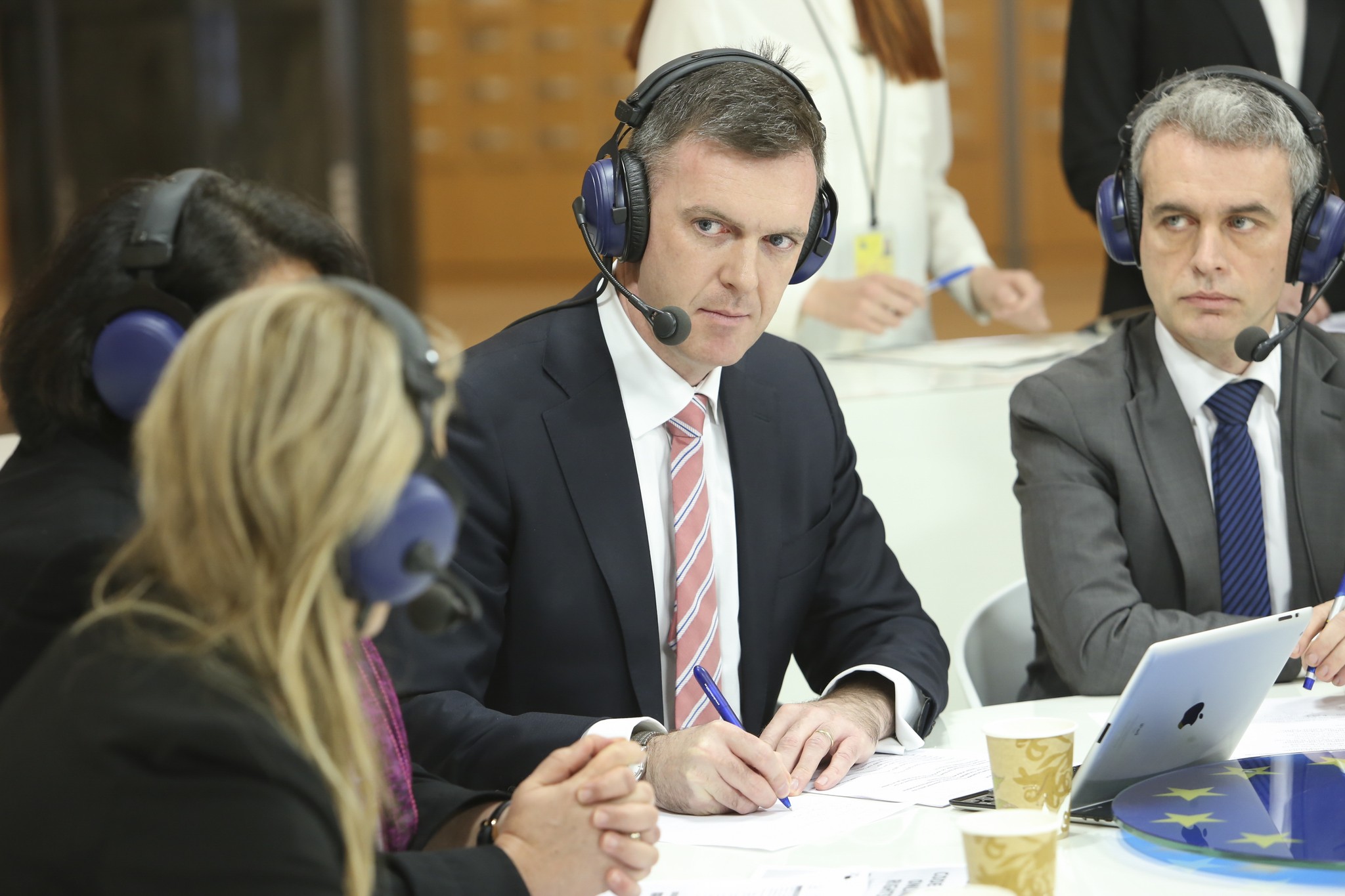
top-left (1301, 574), bottom-right (1345, 691)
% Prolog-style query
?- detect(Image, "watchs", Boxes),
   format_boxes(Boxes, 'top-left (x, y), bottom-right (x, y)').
top-left (624, 731), bottom-right (664, 782)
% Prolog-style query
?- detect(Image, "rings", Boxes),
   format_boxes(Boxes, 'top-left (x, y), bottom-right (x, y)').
top-left (815, 729), bottom-right (833, 748)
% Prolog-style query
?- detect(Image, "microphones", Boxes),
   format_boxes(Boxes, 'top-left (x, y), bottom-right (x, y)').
top-left (1234, 252), bottom-right (1345, 363)
top-left (408, 543), bottom-right (485, 628)
top-left (572, 196), bottom-right (691, 346)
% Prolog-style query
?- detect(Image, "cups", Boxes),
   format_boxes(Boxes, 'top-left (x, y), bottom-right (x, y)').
top-left (983, 720), bottom-right (1076, 842)
top-left (962, 806), bottom-right (1062, 896)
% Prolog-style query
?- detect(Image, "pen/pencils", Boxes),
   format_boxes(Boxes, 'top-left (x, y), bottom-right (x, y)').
top-left (921, 262), bottom-right (974, 297)
top-left (693, 665), bottom-right (794, 813)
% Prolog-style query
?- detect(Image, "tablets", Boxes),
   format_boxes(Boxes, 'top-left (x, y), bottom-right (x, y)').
top-left (1112, 757), bottom-right (1289, 858)
top-left (1064, 606), bottom-right (1315, 816)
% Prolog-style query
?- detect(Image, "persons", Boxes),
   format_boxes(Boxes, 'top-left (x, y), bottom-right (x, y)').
top-left (0, 167), bottom-right (369, 692)
top-left (627, 0), bottom-right (1054, 365)
top-left (1, 274), bottom-right (665, 896)
top-left (1009, 64), bottom-right (1345, 703)
top-left (1060, 0), bottom-right (1345, 328)
top-left (363, 42), bottom-right (948, 811)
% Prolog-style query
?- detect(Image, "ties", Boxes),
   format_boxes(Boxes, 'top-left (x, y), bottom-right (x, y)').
top-left (660, 394), bottom-right (722, 731)
top-left (1203, 379), bottom-right (1271, 618)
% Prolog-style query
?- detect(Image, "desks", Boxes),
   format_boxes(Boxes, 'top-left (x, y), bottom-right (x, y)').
top-left (614, 677), bottom-right (1345, 895)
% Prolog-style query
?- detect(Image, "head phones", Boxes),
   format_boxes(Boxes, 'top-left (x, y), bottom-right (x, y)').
top-left (320, 273), bottom-right (458, 605)
top-left (1099, 65), bottom-right (1345, 282)
top-left (578, 49), bottom-right (838, 285)
top-left (87, 168), bottom-right (234, 438)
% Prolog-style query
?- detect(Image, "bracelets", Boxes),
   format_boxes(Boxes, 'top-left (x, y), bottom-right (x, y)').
top-left (474, 798), bottom-right (511, 849)
